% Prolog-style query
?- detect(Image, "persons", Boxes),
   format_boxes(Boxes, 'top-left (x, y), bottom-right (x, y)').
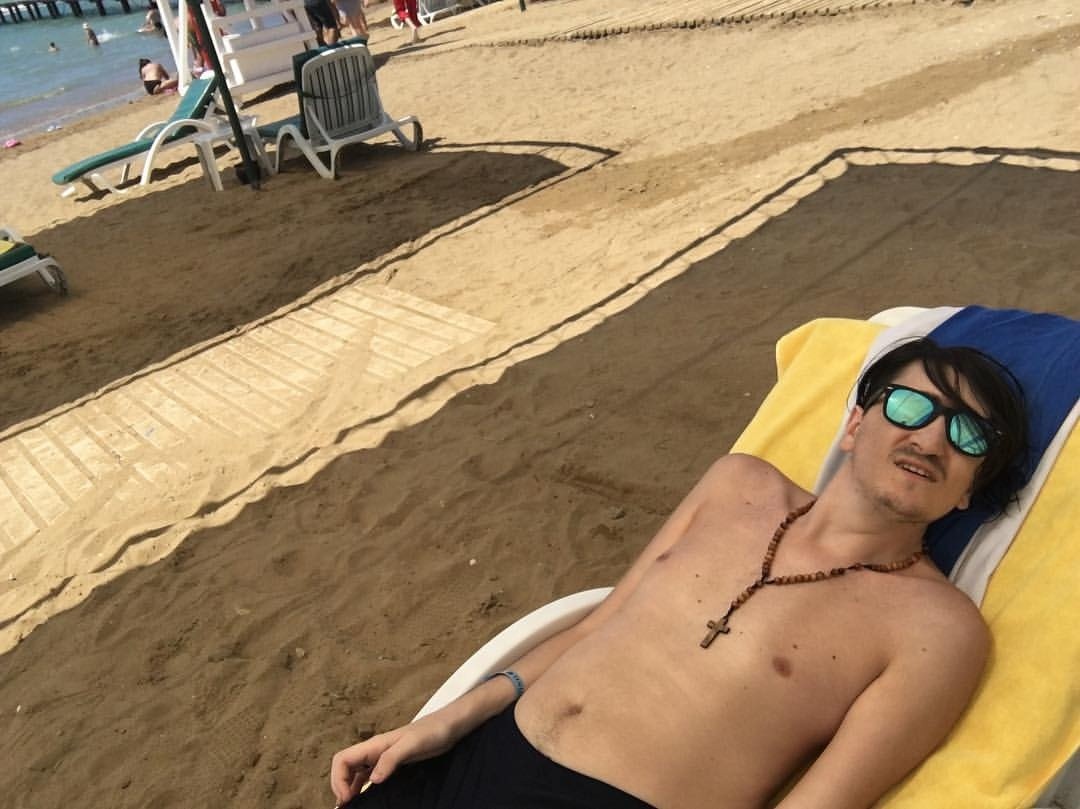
top-left (48, 42), bottom-right (59, 52)
top-left (330, 337), bottom-right (1034, 809)
top-left (304, 0), bottom-right (365, 46)
top-left (82, 22), bottom-right (98, 45)
top-left (393, 0), bottom-right (422, 42)
top-left (147, 3), bottom-right (167, 39)
top-left (139, 58), bottom-right (178, 95)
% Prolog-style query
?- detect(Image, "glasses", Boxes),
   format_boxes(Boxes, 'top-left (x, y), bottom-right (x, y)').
top-left (862, 385), bottom-right (1002, 458)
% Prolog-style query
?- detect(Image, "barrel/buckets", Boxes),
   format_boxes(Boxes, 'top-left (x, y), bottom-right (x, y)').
top-left (234, 159), bottom-right (262, 185)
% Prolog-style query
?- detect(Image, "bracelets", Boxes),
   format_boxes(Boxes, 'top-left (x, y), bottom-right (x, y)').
top-left (488, 671), bottom-right (524, 697)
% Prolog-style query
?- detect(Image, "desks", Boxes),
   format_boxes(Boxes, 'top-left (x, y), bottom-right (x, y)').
top-left (191, 124), bottom-right (260, 190)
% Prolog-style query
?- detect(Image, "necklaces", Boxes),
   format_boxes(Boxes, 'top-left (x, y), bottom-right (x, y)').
top-left (701, 498), bottom-right (928, 649)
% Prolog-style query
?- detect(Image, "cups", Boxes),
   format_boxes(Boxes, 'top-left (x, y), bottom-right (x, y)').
top-left (60, 186), bottom-right (78, 198)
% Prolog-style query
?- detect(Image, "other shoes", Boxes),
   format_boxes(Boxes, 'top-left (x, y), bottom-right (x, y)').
top-left (412, 28), bottom-right (418, 43)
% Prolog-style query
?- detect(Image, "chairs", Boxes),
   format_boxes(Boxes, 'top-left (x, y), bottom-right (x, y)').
top-left (0, 228), bottom-right (67, 297)
top-left (362, 306), bottom-right (1079, 809)
top-left (253, 33), bottom-right (423, 179)
top-left (53, 70), bottom-right (241, 194)
top-left (390, 0), bottom-right (458, 28)
